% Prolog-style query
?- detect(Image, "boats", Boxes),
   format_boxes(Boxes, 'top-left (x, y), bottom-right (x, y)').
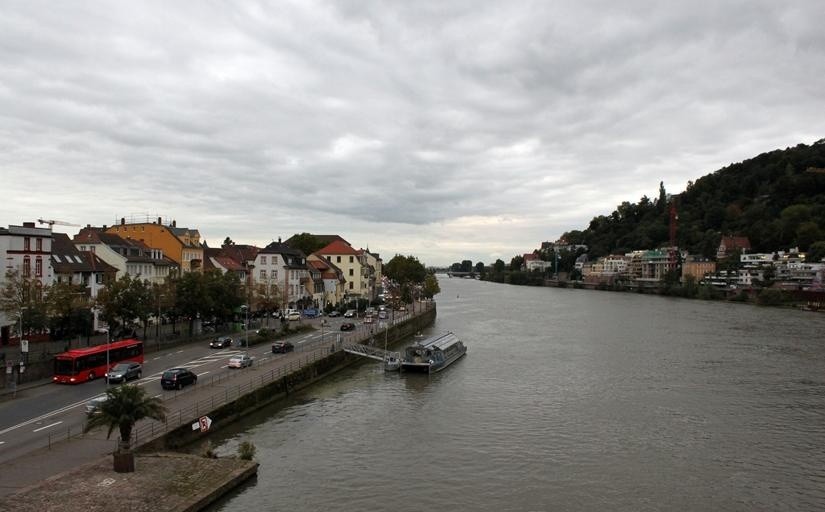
top-left (385, 352), bottom-right (405, 370)
top-left (406, 329), bottom-right (468, 374)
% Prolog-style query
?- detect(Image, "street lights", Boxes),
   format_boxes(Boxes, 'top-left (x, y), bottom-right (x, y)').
top-left (99, 328), bottom-right (109, 400)
top-left (15, 306), bottom-right (29, 384)
top-left (158, 294), bottom-right (165, 349)
top-left (240, 304), bottom-right (248, 374)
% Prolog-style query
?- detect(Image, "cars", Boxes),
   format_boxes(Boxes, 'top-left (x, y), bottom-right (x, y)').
top-left (229, 354), bottom-right (253, 369)
top-left (273, 304), bottom-right (406, 331)
top-left (209, 336), bottom-right (233, 349)
top-left (272, 340), bottom-right (294, 353)
top-left (103, 362), bottom-right (142, 384)
top-left (161, 368), bottom-right (197, 390)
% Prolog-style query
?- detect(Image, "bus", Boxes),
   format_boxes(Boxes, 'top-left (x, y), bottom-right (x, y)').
top-left (53, 338), bottom-right (144, 385)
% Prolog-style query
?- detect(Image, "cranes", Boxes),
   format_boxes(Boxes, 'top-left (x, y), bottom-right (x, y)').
top-left (38, 219), bottom-right (81, 229)
